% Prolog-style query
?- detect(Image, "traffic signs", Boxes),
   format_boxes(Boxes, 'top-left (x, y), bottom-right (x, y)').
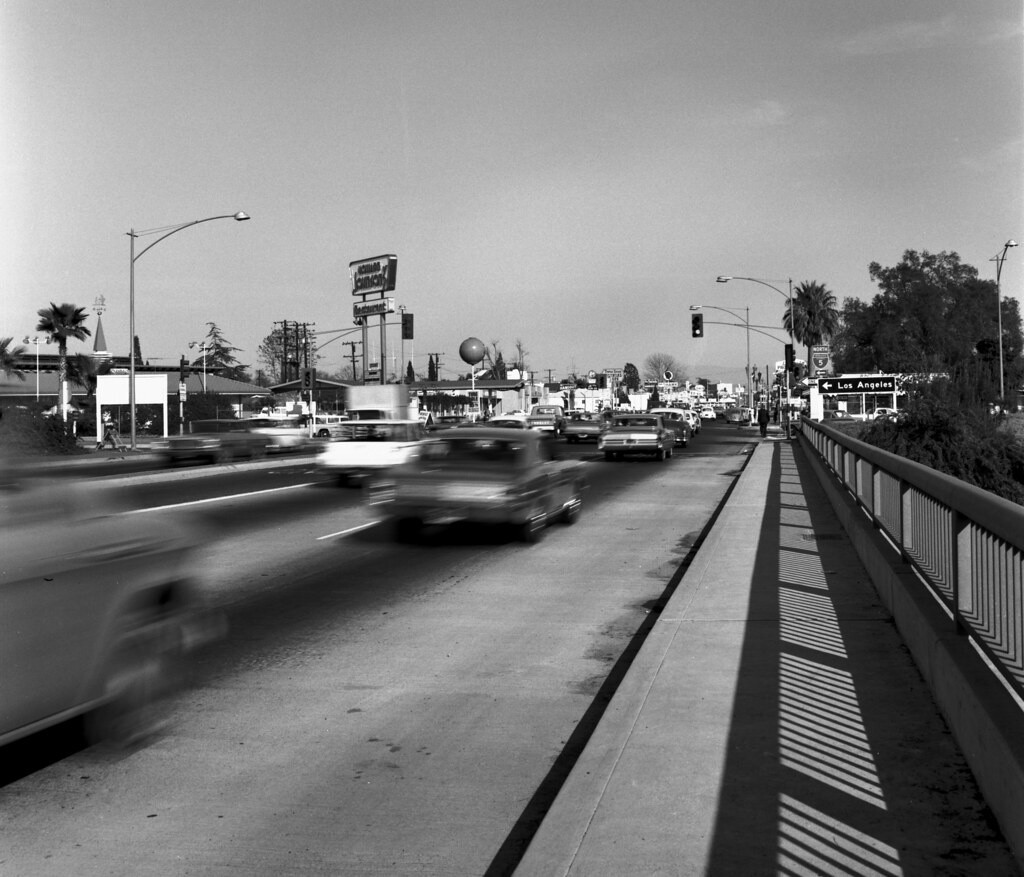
top-left (818, 376), bottom-right (896, 394)
top-left (801, 376), bottom-right (820, 387)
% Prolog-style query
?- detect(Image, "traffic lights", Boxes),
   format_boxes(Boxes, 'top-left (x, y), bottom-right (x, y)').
top-left (691, 313), bottom-right (704, 338)
top-left (301, 368), bottom-right (315, 389)
top-left (785, 344), bottom-right (797, 371)
top-left (793, 363), bottom-right (802, 378)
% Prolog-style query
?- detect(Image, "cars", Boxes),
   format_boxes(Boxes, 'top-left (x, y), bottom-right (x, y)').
top-left (315, 417), bottom-right (440, 477)
top-left (0, 487), bottom-right (231, 759)
top-left (780, 410), bottom-right (853, 437)
top-left (152, 417), bottom-right (309, 466)
top-left (473, 399), bottom-right (727, 461)
top-left (369, 427), bottom-right (596, 545)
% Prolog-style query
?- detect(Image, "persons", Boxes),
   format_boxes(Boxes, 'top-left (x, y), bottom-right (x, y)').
top-left (773, 408), bottom-right (778, 424)
top-left (758, 406), bottom-right (770, 437)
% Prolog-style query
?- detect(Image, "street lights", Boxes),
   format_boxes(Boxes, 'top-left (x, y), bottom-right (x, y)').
top-left (688, 303), bottom-right (751, 407)
top-left (716, 275), bottom-right (800, 421)
top-left (22, 334), bottom-right (53, 401)
top-left (989, 238), bottom-right (1019, 400)
top-left (125, 211), bottom-right (251, 452)
top-left (397, 304), bottom-right (408, 385)
top-left (750, 372), bottom-right (762, 423)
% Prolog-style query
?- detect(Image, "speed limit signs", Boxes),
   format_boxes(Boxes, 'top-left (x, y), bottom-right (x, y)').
top-left (811, 345), bottom-right (830, 370)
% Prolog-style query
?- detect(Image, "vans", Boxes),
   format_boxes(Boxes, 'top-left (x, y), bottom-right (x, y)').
top-left (307, 415), bottom-right (350, 437)
